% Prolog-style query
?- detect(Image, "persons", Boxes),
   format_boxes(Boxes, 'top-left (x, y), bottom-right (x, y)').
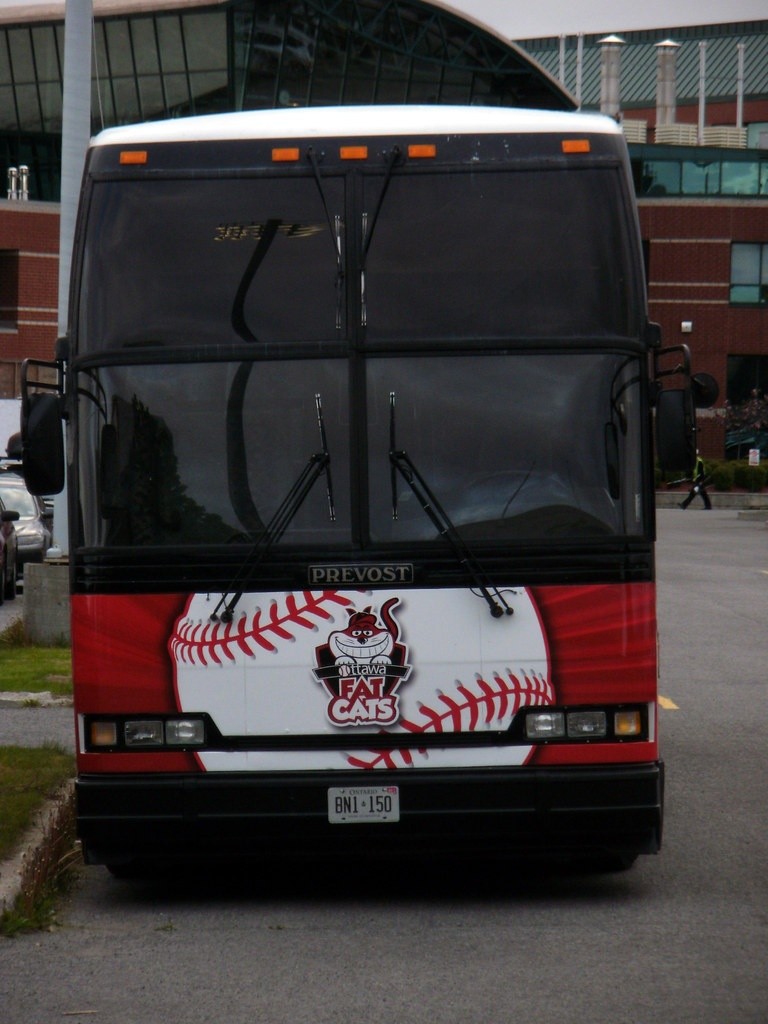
top-left (677, 449), bottom-right (712, 510)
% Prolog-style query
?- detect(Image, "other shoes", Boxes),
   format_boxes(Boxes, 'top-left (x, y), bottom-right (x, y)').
top-left (677, 502), bottom-right (685, 510)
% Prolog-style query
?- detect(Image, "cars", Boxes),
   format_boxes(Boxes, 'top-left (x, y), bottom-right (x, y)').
top-left (0, 459), bottom-right (53, 606)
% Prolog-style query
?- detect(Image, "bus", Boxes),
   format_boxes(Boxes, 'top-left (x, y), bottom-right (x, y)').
top-left (20, 101), bottom-right (721, 893)
